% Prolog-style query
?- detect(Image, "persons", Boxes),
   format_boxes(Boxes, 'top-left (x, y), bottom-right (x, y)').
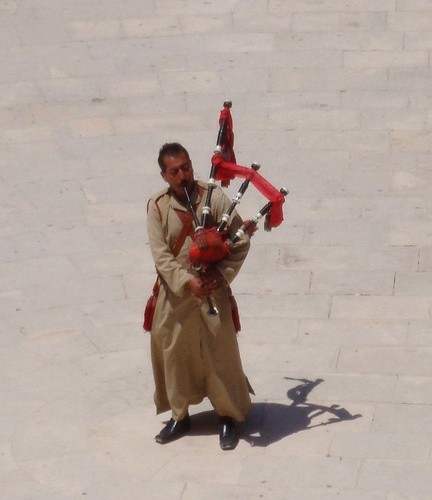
top-left (143, 140), bottom-right (256, 451)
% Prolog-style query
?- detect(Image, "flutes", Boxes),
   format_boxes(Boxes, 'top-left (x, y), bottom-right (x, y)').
top-left (182, 100), bottom-right (287, 318)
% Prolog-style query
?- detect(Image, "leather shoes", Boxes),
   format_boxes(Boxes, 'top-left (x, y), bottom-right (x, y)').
top-left (156, 420), bottom-right (191, 443)
top-left (219, 419), bottom-right (239, 451)
